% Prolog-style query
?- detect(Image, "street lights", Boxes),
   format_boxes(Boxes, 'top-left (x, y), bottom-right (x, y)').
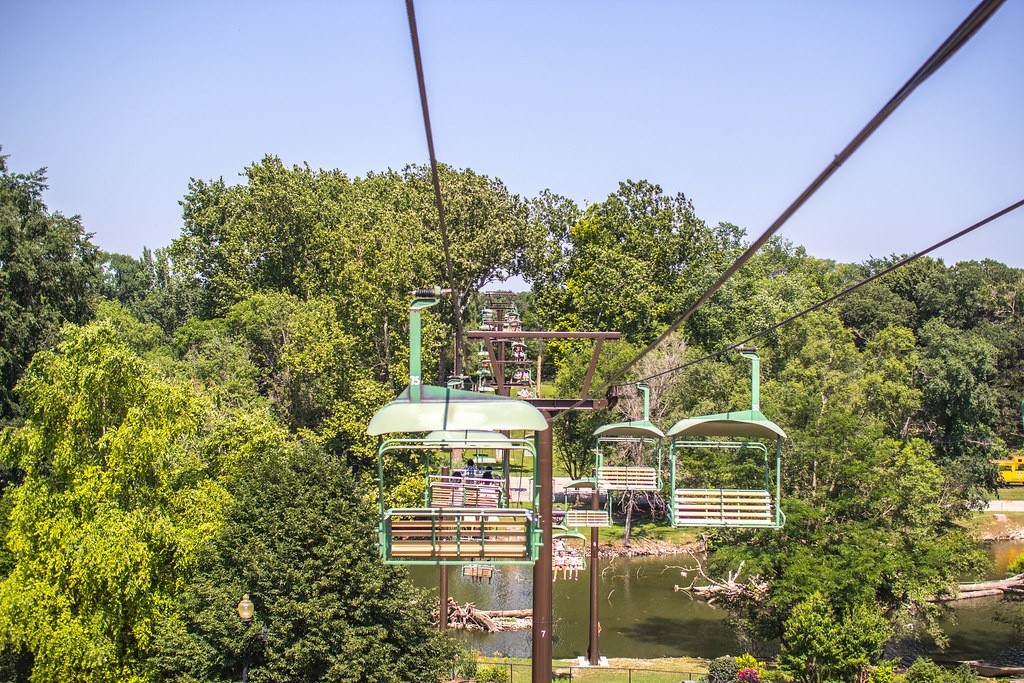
top-left (238, 592), bottom-right (269, 683)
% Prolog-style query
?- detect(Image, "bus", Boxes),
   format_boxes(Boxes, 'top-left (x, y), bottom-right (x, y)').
top-left (987, 455), bottom-right (1024, 486)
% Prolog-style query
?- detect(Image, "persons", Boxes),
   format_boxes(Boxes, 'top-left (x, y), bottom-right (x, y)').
top-left (514, 350), bottom-right (525, 361)
top-left (516, 369), bottom-right (529, 384)
top-left (447, 472), bottom-right (465, 521)
top-left (460, 459), bottom-right (479, 483)
top-left (520, 387), bottom-right (534, 399)
top-left (475, 473), bottom-right (497, 521)
top-left (482, 378), bottom-right (488, 387)
top-left (511, 326), bottom-right (519, 332)
top-left (486, 466), bottom-right (495, 479)
top-left (509, 315), bottom-right (516, 321)
